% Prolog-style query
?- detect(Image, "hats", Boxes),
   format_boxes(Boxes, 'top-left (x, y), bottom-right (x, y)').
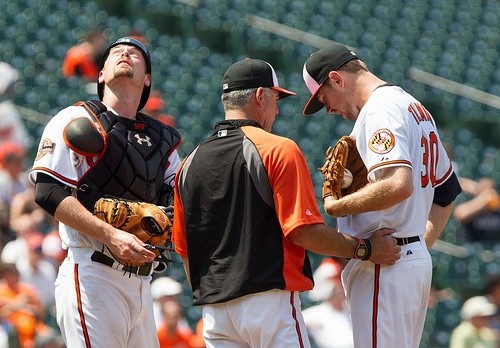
top-left (97, 37), bottom-right (152, 112)
top-left (0, 62), bottom-right (20, 98)
top-left (222, 58), bottom-right (297, 100)
top-left (303, 43), bottom-right (358, 116)
top-left (461, 296), bottom-right (497, 319)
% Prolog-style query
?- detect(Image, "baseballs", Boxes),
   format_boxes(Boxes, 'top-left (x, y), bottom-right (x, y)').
top-left (340, 169), bottom-right (352, 189)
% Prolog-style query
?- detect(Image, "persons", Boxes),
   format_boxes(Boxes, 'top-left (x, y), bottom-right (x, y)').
top-left (0, 62), bottom-right (68, 347)
top-left (170, 57), bottom-right (401, 348)
top-left (449, 279), bottom-right (500, 348)
top-left (28, 37), bottom-right (182, 348)
top-left (302, 257), bottom-right (354, 348)
top-left (302, 44), bottom-right (462, 348)
top-left (151, 277), bottom-right (206, 348)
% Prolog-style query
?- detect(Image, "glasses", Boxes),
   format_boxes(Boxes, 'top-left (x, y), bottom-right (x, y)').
top-left (134, 244), bottom-right (183, 265)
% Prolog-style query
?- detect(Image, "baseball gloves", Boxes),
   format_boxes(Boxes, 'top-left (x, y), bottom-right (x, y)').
top-left (93, 196), bottom-right (173, 278)
top-left (317, 136), bottom-right (370, 217)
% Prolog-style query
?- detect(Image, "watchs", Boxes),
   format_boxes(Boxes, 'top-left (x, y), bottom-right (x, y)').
top-left (355, 238), bottom-right (368, 260)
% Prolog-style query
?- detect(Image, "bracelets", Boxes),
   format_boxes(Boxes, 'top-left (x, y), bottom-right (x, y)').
top-left (362, 239), bottom-right (371, 261)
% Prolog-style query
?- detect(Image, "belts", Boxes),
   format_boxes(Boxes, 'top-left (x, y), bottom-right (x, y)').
top-left (65, 247), bottom-right (153, 278)
top-left (391, 236), bottom-right (420, 245)
